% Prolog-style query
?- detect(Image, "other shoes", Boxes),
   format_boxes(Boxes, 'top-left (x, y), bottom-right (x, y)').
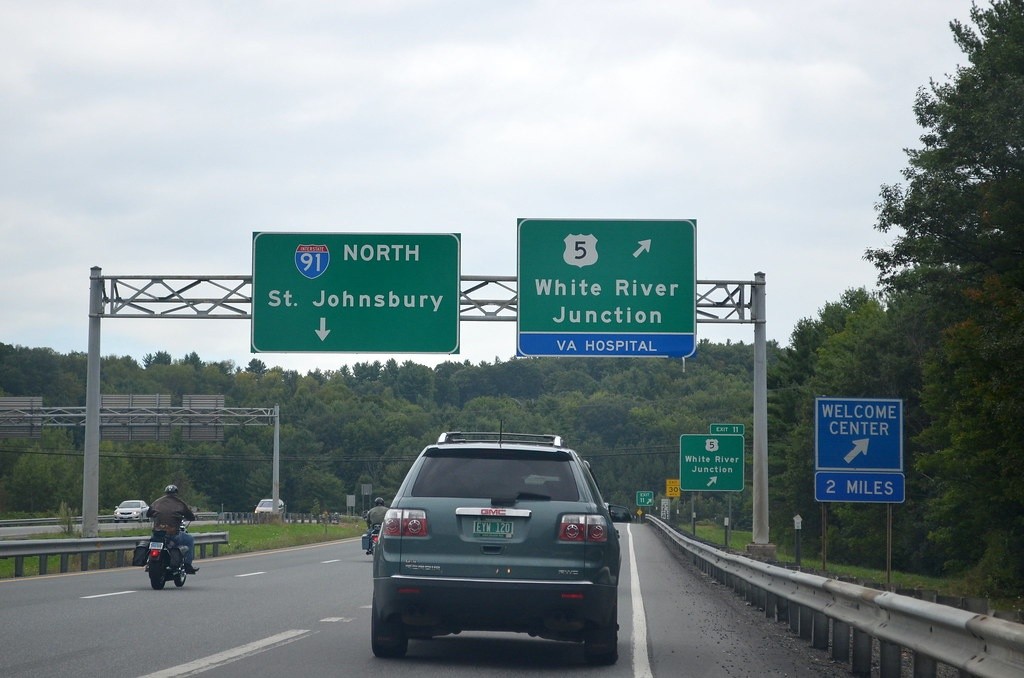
top-left (183, 563), bottom-right (199, 574)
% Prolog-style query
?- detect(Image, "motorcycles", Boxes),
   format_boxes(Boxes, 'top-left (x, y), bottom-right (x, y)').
top-left (362, 509), bottom-right (382, 560)
top-left (131, 506), bottom-right (203, 590)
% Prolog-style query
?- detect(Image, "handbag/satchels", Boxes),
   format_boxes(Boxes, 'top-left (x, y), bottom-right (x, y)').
top-left (133, 539), bottom-right (150, 567)
top-left (361, 533), bottom-right (370, 549)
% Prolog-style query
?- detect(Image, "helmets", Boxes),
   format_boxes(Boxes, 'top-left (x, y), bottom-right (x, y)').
top-left (374, 497), bottom-right (384, 507)
top-left (163, 484), bottom-right (179, 496)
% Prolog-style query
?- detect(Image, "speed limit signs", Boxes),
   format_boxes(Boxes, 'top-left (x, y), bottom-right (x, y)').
top-left (666, 480), bottom-right (681, 496)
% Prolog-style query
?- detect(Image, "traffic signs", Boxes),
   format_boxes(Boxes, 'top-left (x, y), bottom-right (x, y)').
top-left (516, 216), bottom-right (698, 359)
top-left (250, 232), bottom-right (462, 355)
top-left (814, 396), bottom-right (904, 472)
top-left (680, 432), bottom-right (746, 492)
top-left (636, 490), bottom-right (654, 507)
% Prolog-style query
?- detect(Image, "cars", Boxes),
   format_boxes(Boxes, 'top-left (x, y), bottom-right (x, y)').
top-left (370, 430), bottom-right (621, 667)
top-left (113, 499), bottom-right (151, 522)
top-left (255, 499), bottom-right (285, 514)
top-left (604, 502), bottom-right (634, 523)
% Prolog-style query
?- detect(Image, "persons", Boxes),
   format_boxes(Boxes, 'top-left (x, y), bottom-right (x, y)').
top-left (147, 485), bottom-right (200, 574)
top-left (365, 497), bottom-right (390, 556)
